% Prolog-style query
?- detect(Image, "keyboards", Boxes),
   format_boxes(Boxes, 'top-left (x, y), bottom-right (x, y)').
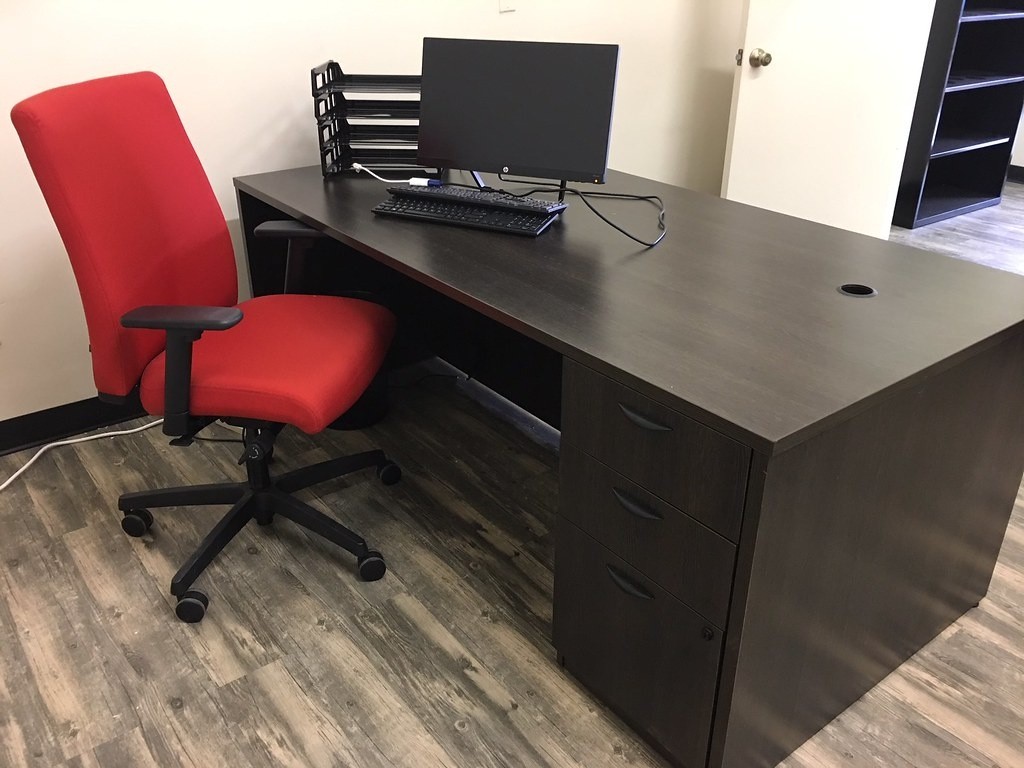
top-left (370, 183), bottom-right (569, 237)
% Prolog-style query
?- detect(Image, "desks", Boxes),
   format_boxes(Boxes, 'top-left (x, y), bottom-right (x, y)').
top-left (230, 148), bottom-right (1024, 767)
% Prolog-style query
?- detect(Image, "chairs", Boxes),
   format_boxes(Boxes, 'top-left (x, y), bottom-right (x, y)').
top-left (9, 71), bottom-right (400, 627)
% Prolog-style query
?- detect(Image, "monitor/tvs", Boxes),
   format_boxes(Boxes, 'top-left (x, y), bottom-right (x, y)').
top-left (417, 37), bottom-right (619, 184)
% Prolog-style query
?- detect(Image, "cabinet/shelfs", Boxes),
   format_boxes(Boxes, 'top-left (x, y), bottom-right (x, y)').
top-left (889, 0), bottom-right (1024, 230)
top-left (552, 356), bottom-right (753, 768)
